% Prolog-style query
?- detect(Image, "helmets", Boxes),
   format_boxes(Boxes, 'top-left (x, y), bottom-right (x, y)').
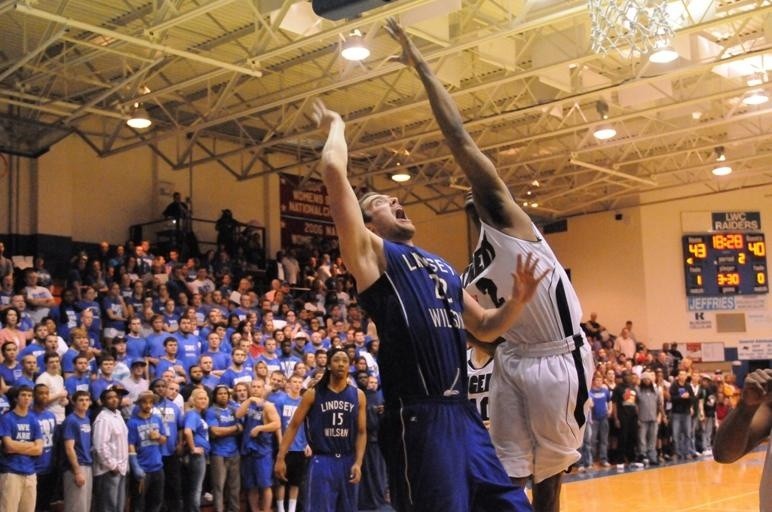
top-left (111, 334), bottom-right (129, 344)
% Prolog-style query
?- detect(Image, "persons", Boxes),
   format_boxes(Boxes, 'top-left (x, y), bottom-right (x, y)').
top-left (711, 365), bottom-right (771, 511)
top-left (214, 208), bottom-right (240, 260)
top-left (162, 192), bottom-right (188, 229)
top-left (377, 14), bottom-right (598, 511)
top-left (306, 97), bottom-right (554, 509)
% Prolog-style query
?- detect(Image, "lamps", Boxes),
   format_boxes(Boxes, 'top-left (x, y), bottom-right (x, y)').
top-left (649, 34), bottom-right (679, 64)
top-left (339, 13), bottom-right (371, 61)
top-left (743, 74), bottom-right (769, 106)
top-left (127, 100), bottom-right (152, 128)
top-left (391, 163), bottom-right (411, 182)
top-left (593, 99), bottom-right (617, 139)
top-left (711, 146), bottom-right (732, 178)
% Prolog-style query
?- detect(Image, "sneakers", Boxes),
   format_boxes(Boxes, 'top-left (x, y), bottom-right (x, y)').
top-left (566, 444), bottom-right (715, 474)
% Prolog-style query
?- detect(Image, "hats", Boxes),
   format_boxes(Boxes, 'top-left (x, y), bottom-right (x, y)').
top-left (131, 391), bottom-right (160, 406)
top-left (106, 384), bottom-right (128, 397)
top-left (293, 331), bottom-right (307, 340)
top-left (129, 356), bottom-right (146, 368)
